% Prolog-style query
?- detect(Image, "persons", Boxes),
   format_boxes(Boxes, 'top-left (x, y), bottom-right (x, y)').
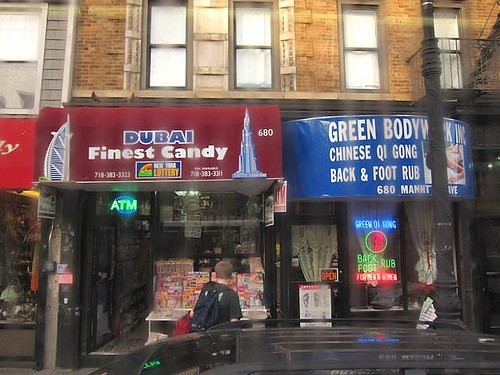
top-left (426, 144), bottom-right (465, 183)
top-left (189, 260), bottom-right (243, 334)
